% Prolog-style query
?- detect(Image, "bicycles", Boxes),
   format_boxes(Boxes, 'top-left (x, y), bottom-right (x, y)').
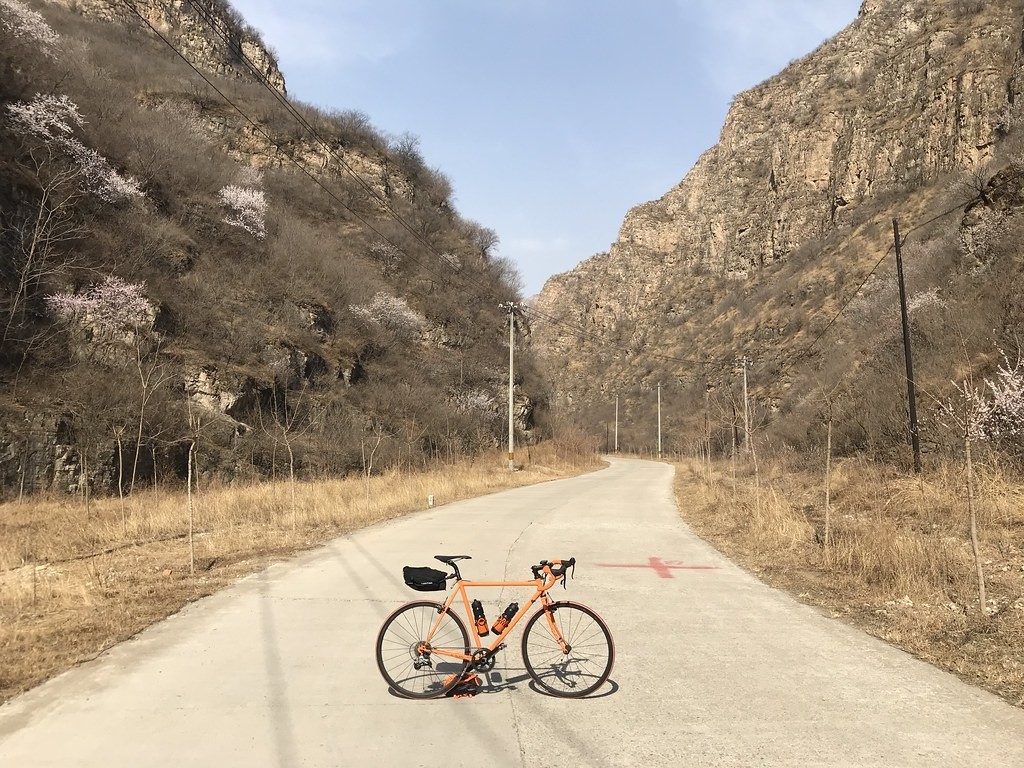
top-left (377, 556), bottom-right (614, 700)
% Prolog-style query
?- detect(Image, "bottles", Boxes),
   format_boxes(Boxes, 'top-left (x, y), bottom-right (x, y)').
top-left (471, 599), bottom-right (489, 637)
top-left (491, 602), bottom-right (519, 635)
